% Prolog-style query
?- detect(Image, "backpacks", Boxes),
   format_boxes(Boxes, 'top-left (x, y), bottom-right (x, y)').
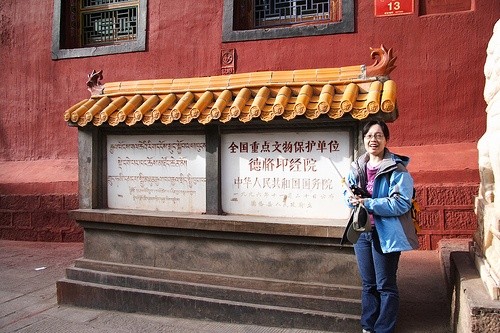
top-left (387, 162), bottom-right (420, 235)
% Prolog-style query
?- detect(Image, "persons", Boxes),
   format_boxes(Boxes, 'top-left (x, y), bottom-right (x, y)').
top-left (342, 119), bottom-right (420, 333)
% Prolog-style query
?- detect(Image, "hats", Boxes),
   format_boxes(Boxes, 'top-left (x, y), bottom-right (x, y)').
top-left (346, 202), bottom-right (370, 245)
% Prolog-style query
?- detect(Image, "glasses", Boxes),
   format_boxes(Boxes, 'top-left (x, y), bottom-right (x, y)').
top-left (364, 133), bottom-right (383, 139)
